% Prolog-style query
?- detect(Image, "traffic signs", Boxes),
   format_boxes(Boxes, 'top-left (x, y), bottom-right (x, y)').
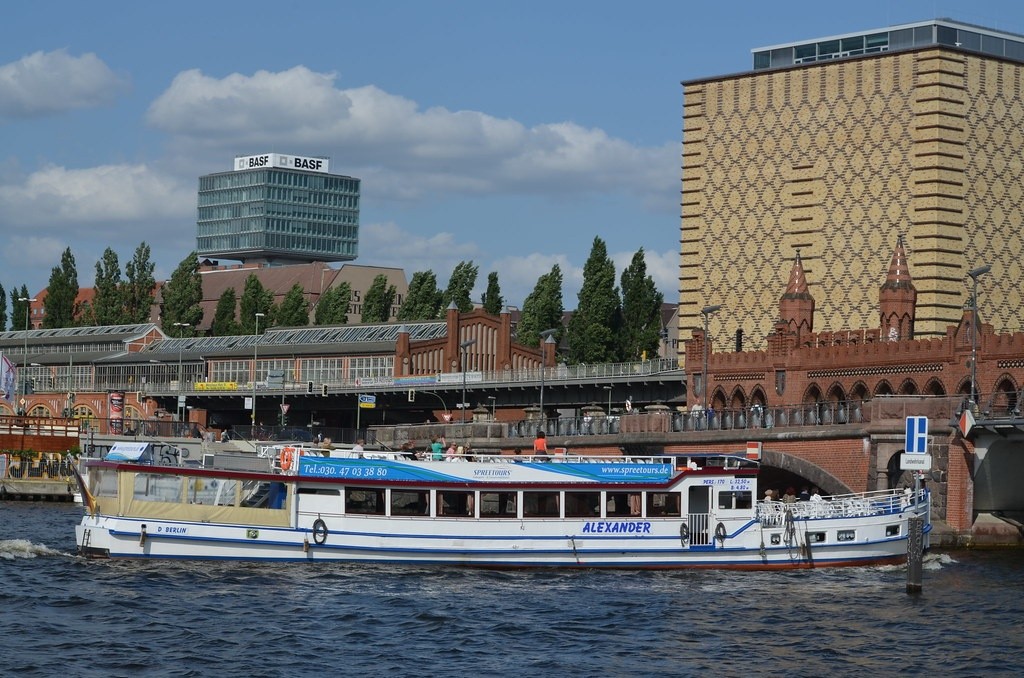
top-left (359, 403), bottom-right (376, 408)
top-left (358, 395), bottom-right (378, 405)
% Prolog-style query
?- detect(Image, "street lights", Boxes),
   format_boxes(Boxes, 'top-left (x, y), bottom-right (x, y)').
top-left (254, 313), bottom-right (265, 440)
top-left (488, 396), bottom-right (496, 421)
top-left (172, 322), bottom-right (191, 436)
top-left (700, 305), bottom-right (725, 418)
top-left (603, 386), bottom-right (614, 434)
top-left (536, 328), bottom-right (559, 419)
top-left (30, 362), bottom-right (73, 439)
top-left (970, 265), bottom-right (997, 417)
top-left (460, 337), bottom-right (477, 427)
top-left (18, 297), bottom-right (42, 419)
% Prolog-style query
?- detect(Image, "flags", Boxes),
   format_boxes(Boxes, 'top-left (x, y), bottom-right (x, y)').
top-left (1, 356), bottom-right (16, 402)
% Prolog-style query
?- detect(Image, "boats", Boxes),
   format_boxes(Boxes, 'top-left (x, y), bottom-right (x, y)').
top-left (65, 411), bottom-right (930, 568)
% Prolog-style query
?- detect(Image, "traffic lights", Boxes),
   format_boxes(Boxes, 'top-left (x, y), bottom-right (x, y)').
top-left (408, 389), bottom-right (416, 404)
top-left (322, 384), bottom-right (329, 397)
top-left (307, 381), bottom-right (313, 395)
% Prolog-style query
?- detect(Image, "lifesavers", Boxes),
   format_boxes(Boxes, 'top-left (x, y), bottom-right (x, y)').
top-left (281, 448), bottom-right (292, 471)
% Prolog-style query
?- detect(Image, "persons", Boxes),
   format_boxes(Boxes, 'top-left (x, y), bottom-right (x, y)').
top-left (191, 423), bottom-right (204, 440)
top-left (691, 400), bottom-right (702, 418)
top-left (221, 429), bottom-right (230, 442)
top-left (704, 403), bottom-right (715, 422)
top-left (424, 434), bottom-right (446, 461)
top-left (782, 487), bottom-right (796, 503)
top-left (353, 438), bottom-right (365, 459)
top-left (257, 421), bottom-right (267, 435)
top-left (400, 439), bottom-right (420, 461)
top-left (312, 437), bottom-right (336, 457)
top-left (513, 448), bottom-right (522, 462)
top-left (800, 487), bottom-right (823, 502)
top-left (534, 431), bottom-right (551, 461)
top-left (445, 441), bottom-right (473, 462)
top-left (904, 482), bottom-right (913, 497)
top-left (765, 487), bottom-right (779, 501)
